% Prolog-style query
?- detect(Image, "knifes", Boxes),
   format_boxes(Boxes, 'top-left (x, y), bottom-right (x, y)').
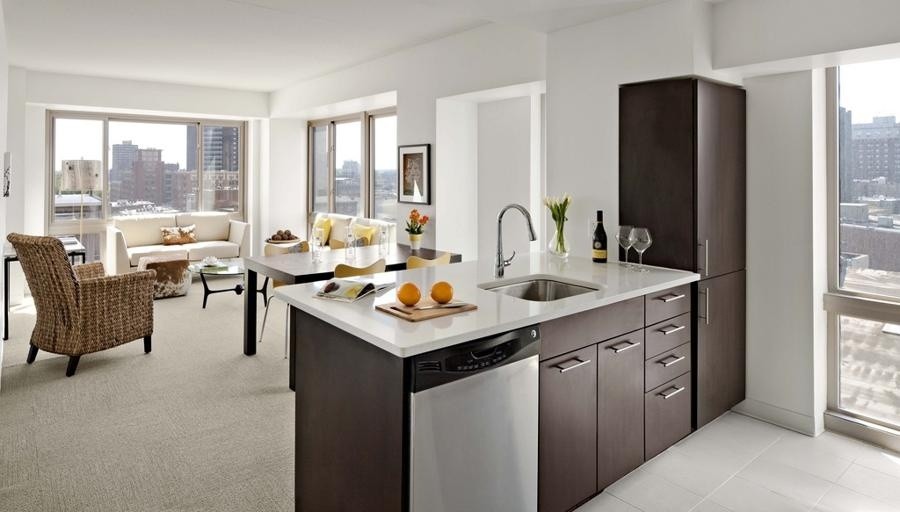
top-left (413, 303), bottom-right (469, 309)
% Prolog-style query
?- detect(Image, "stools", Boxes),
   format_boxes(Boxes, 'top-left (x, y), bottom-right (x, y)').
top-left (138, 256), bottom-right (190, 300)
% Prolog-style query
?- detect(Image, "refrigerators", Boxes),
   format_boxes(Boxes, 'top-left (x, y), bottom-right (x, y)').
top-left (618, 74), bottom-right (747, 431)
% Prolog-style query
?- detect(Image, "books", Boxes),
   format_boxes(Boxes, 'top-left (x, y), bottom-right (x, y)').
top-left (195, 261), bottom-right (229, 272)
top-left (312, 276), bottom-right (397, 304)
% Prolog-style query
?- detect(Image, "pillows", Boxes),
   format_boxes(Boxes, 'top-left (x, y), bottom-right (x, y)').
top-left (312, 218), bottom-right (330, 245)
top-left (180, 223), bottom-right (198, 244)
top-left (160, 226), bottom-right (179, 245)
top-left (352, 223), bottom-right (375, 247)
top-left (330, 216), bottom-right (351, 247)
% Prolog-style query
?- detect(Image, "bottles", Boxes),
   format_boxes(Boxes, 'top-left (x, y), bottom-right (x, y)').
top-left (591, 209), bottom-right (607, 263)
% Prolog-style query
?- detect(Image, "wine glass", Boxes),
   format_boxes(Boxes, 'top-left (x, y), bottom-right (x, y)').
top-left (628, 227), bottom-right (652, 271)
top-left (615, 225), bottom-right (634, 267)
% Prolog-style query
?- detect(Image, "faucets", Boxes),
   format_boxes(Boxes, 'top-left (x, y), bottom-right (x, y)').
top-left (493, 202), bottom-right (537, 281)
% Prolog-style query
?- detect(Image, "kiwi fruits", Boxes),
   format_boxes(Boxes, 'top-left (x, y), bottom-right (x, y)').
top-left (271, 230), bottom-right (295, 240)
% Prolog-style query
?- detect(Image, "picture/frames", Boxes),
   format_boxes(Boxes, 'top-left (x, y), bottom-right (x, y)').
top-left (398, 143), bottom-right (432, 206)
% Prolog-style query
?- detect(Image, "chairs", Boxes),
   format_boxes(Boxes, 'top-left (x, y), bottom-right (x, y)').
top-left (334, 258), bottom-right (386, 278)
top-left (7, 232), bottom-right (157, 377)
top-left (405, 252), bottom-right (451, 270)
top-left (260, 241), bottom-right (309, 359)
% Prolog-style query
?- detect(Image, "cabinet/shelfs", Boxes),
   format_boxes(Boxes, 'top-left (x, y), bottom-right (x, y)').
top-left (612, 73), bottom-right (749, 282)
top-left (695, 268), bottom-right (746, 431)
top-left (538, 297), bottom-right (646, 512)
top-left (646, 284), bottom-right (695, 465)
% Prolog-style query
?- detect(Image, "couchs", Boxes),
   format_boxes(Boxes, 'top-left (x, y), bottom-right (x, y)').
top-left (106, 211), bottom-right (251, 275)
top-left (311, 212), bottom-right (396, 251)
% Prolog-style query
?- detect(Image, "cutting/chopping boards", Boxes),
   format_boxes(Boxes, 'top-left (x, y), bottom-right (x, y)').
top-left (375, 296), bottom-right (477, 321)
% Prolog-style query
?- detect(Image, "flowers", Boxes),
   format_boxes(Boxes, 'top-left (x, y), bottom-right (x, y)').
top-left (542, 193), bottom-right (570, 253)
top-left (403, 208), bottom-right (428, 233)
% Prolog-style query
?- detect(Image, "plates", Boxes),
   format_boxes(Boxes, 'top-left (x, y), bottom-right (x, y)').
top-left (266, 237), bottom-right (301, 244)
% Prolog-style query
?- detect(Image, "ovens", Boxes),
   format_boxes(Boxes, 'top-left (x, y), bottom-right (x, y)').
top-left (407, 324), bottom-right (540, 512)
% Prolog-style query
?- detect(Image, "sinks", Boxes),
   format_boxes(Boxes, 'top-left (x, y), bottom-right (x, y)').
top-left (475, 271), bottom-right (609, 306)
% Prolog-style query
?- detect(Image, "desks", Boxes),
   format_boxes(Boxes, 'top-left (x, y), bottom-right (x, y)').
top-left (243, 243), bottom-right (462, 394)
top-left (263, 235), bottom-right (301, 244)
top-left (1, 237), bottom-right (87, 342)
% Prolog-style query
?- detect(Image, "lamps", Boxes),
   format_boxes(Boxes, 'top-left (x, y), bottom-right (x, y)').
top-left (4, 152), bottom-right (11, 198)
top-left (61, 158), bottom-right (104, 245)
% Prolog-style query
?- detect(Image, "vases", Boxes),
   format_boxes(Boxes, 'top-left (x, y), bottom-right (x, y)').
top-left (408, 233), bottom-right (423, 249)
top-left (549, 219), bottom-right (570, 258)
